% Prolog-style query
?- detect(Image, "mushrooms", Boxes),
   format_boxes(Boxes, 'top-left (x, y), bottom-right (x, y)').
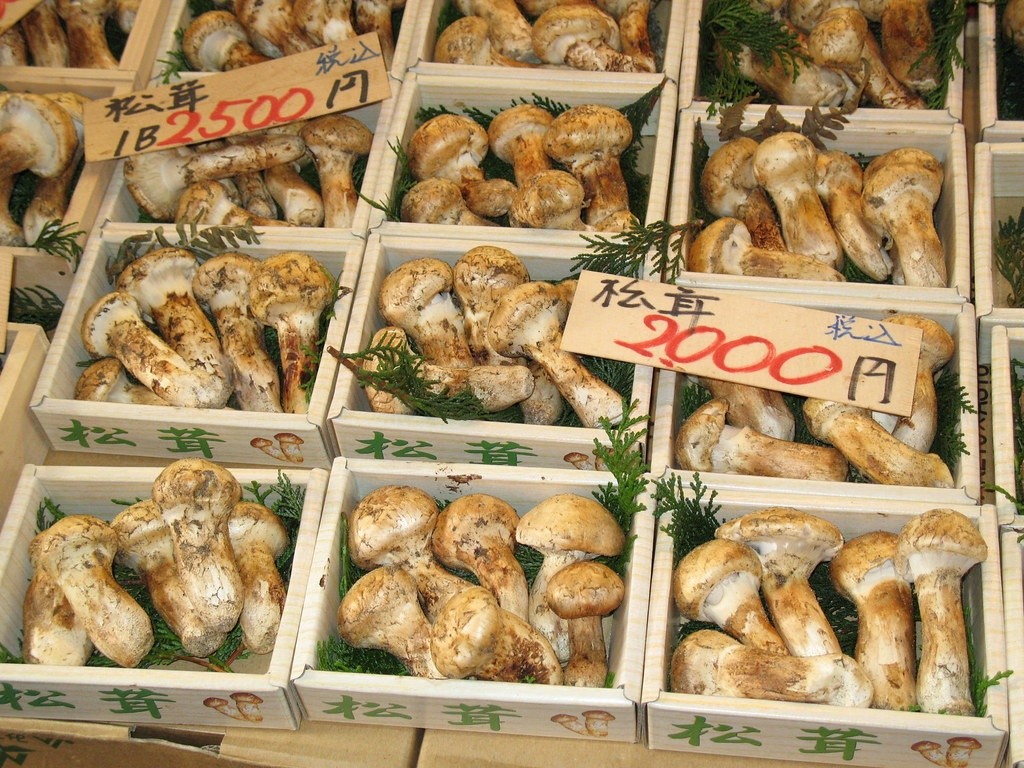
top-left (3, 2), bottom-right (989, 714)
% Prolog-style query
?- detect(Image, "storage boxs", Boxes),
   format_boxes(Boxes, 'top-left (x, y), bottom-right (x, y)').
top-left (0, 0), bottom-right (1024, 768)
